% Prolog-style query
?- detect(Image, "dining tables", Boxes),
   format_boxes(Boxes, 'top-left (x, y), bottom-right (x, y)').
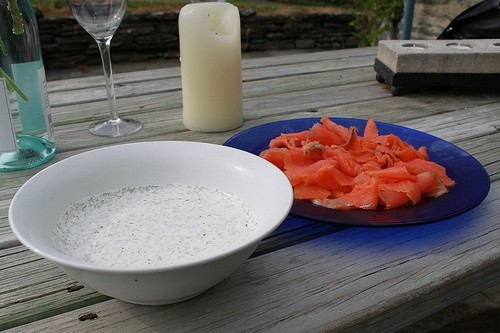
top-left (1, 43), bottom-right (499, 332)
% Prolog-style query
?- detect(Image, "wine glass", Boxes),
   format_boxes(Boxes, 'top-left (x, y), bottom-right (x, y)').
top-left (67, 0), bottom-right (144, 137)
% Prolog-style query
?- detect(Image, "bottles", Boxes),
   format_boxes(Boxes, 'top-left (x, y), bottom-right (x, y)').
top-left (0, 0), bottom-right (57, 173)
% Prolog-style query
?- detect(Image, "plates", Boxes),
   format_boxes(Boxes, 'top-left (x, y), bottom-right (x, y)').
top-left (221, 117), bottom-right (491, 227)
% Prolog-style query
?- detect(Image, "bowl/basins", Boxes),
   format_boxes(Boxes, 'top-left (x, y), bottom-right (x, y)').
top-left (7, 141), bottom-right (294, 306)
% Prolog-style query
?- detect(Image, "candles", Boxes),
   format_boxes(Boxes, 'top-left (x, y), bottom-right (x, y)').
top-left (178, 1), bottom-right (246, 134)
